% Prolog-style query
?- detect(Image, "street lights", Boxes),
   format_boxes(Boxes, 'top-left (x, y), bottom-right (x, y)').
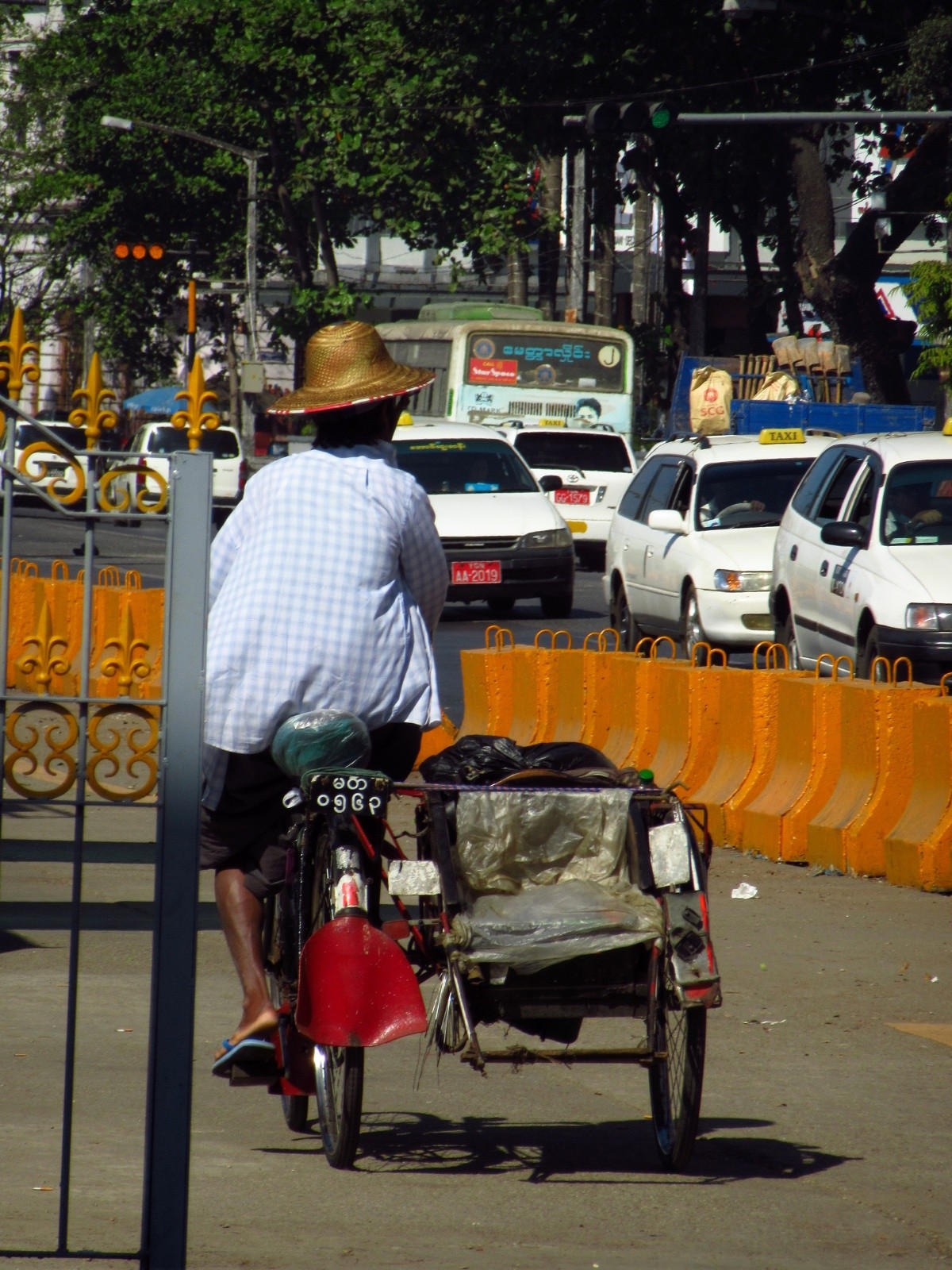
top-left (101, 114), bottom-right (261, 444)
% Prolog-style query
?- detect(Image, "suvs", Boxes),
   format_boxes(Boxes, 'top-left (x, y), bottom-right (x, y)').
top-left (103, 422), bottom-right (250, 530)
top-left (606, 429), bottom-right (855, 666)
top-left (489, 419), bottom-right (638, 565)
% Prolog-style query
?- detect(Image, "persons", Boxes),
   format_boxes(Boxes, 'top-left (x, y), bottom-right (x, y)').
top-left (883, 483), bottom-right (942, 543)
top-left (468, 456), bottom-right (489, 484)
top-left (699, 475), bottom-right (766, 527)
top-left (196, 322), bottom-right (447, 1076)
top-left (575, 398), bottom-right (614, 432)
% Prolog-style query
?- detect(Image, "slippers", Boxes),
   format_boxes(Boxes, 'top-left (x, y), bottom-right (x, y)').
top-left (210, 1034), bottom-right (275, 1079)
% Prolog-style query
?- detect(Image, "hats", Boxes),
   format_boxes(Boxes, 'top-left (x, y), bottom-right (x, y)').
top-left (266, 321), bottom-right (437, 417)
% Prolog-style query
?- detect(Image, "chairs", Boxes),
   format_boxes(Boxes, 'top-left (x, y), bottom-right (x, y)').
top-left (839, 471), bottom-right (936, 536)
top-left (668, 472), bottom-right (794, 525)
top-left (403, 445), bottom-right (508, 496)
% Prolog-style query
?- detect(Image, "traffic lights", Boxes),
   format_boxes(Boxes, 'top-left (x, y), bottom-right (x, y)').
top-left (585, 99), bottom-right (679, 133)
top-left (110, 242), bottom-right (163, 261)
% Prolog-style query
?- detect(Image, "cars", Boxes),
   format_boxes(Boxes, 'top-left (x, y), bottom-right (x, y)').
top-left (1, 418), bottom-right (107, 502)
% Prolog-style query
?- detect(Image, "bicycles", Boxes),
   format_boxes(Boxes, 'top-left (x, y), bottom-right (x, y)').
top-left (226, 709), bottom-right (726, 1171)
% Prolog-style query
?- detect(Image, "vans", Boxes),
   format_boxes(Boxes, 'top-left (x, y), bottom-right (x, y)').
top-left (382, 414), bottom-right (579, 624)
top-left (771, 418), bottom-right (952, 686)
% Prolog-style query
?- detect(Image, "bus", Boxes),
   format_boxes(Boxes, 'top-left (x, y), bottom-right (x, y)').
top-left (378, 301), bottom-right (633, 467)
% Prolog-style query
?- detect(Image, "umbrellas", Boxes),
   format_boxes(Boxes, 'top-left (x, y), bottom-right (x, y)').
top-left (123, 386), bottom-right (224, 417)
